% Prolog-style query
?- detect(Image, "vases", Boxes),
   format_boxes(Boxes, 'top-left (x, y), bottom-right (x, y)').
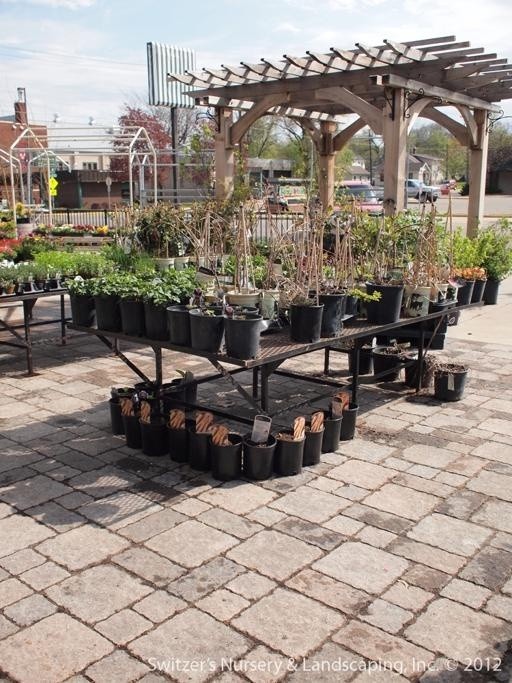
top-left (109, 377), bottom-right (359, 481)
top-left (348, 344), bottom-right (470, 400)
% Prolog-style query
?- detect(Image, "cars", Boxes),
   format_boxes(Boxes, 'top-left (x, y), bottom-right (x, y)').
top-left (336, 180), bottom-right (384, 217)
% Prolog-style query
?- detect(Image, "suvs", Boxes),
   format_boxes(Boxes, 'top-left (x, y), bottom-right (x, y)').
top-left (405, 179), bottom-right (440, 202)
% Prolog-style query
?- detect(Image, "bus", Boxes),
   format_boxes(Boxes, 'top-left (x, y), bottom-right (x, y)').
top-left (267, 178), bottom-right (316, 204)
